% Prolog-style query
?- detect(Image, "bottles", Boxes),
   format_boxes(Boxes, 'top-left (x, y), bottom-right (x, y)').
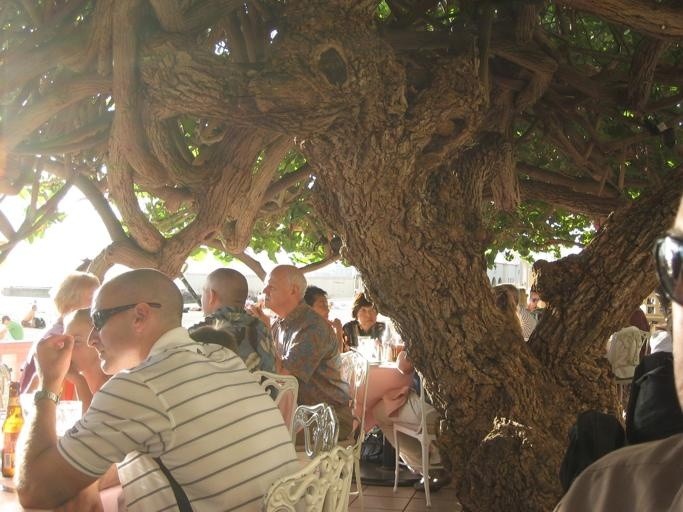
top-left (1, 380), bottom-right (25, 478)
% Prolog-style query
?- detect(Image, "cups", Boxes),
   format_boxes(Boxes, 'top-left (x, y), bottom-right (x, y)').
top-left (55, 400), bottom-right (82, 438)
top-left (357, 336), bottom-right (401, 363)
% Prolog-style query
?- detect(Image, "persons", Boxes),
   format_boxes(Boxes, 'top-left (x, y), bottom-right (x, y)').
top-left (1, 263), bottom-right (450, 512)
top-left (499, 258), bottom-right (550, 344)
top-left (552, 197), bottom-right (682, 512)
top-left (630, 309), bottom-right (652, 361)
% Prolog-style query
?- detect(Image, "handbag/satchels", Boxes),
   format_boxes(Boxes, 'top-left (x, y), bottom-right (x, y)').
top-left (351, 429), bottom-right (419, 487)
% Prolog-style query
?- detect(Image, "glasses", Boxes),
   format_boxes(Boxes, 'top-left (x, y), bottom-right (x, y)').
top-left (655, 231), bottom-right (683, 307)
top-left (89, 303), bottom-right (162, 330)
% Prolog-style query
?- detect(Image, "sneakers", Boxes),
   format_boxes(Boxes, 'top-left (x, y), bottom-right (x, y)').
top-left (413, 469), bottom-right (452, 492)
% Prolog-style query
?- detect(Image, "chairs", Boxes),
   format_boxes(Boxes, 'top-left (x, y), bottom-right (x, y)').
top-left (251, 346), bottom-right (441, 511)
top-left (606, 327), bottom-right (651, 404)
